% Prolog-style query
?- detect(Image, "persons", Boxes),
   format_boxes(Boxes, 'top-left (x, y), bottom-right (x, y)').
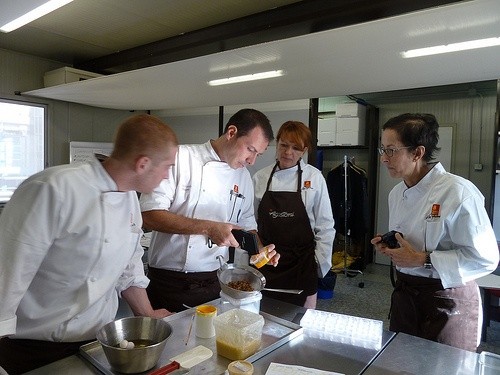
top-left (140, 108), bottom-right (281, 311)
top-left (370, 113), bottom-right (499, 353)
top-left (251, 121), bottom-right (336, 311)
top-left (0, 114), bottom-right (178, 375)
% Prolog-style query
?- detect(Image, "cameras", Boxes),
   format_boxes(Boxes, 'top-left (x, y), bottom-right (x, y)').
top-left (380, 230), bottom-right (403, 249)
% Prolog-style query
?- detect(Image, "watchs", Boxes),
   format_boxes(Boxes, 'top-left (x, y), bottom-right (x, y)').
top-left (422, 252), bottom-right (433, 270)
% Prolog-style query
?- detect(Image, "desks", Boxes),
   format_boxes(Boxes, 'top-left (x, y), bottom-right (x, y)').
top-left (35, 296), bottom-right (500, 375)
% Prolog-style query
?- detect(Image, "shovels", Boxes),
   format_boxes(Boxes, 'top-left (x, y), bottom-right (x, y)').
top-left (145, 344), bottom-right (214, 375)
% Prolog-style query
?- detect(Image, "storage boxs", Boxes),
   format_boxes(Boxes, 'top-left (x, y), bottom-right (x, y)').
top-left (43, 67), bottom-right (103, 89)
top-left (337, 117), bottom-right (366, 132)
top-left (336, 131), bottom-right (365, 146)
top-left (213, 308), bottom-right (265, 361)
top-left (318, 118), bottom-right (337, 132)
top-left (336, 103), bottom-right (367, 119)
top-left (301, 308), bottom-right (384, 350)
top-left (220, 290), bottom-right (263, 314)
top-left (318, 132), bottom-right (336, 146)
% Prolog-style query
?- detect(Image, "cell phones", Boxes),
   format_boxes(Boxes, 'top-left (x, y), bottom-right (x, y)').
top-left (231, 229), bottom-right (259, 255)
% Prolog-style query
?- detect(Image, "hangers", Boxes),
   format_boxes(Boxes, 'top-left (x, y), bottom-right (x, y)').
top-left (327, 158), bottom-right (366, 174)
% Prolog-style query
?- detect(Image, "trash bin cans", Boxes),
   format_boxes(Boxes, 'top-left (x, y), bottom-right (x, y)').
top-left (317, 271), bottom-right (337, 299)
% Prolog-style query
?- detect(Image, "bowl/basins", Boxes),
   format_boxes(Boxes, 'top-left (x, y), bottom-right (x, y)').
top-left (96, 316), bottom-right (173, 374)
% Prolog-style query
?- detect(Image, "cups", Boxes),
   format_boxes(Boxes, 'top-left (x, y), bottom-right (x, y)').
top-left (228, 360), bottom-right (254, 375)
top-left (195, 305), bottom-right (217, 339)
